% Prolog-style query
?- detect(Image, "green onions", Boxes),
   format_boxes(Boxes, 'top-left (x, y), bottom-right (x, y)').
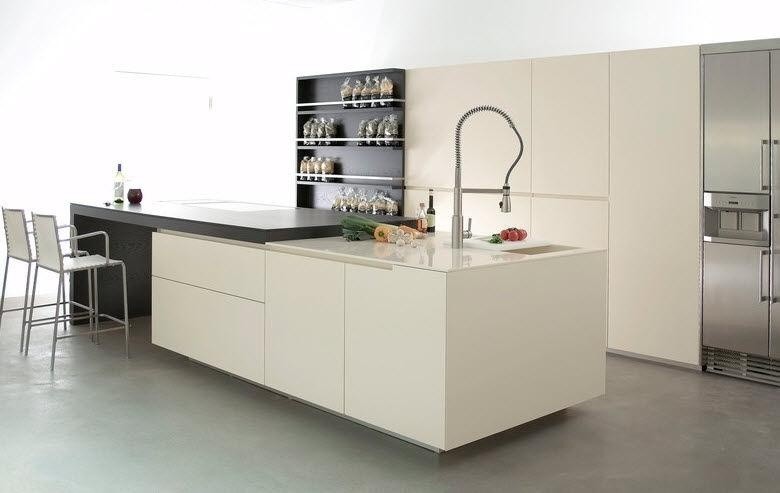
top-left (341, 214), bottom-right (399, 235)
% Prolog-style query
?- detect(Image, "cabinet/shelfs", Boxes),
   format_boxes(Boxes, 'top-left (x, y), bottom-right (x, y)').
top-left (292, 65), bottom-right (409, 220)
top-left (399, 56), bottom-right (533, 245)
top-left (605, 42), bottom-right (704, 377)
top-left (259, 242), bottom-right (608, 456)
top-left (148, 225), bottom-right (268, 395)
top-left (531, 50), bottom-right (609, 249)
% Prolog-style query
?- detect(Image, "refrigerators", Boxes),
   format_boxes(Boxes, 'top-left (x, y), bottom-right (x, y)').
top-left (703, 38), bottom-right (780, 387)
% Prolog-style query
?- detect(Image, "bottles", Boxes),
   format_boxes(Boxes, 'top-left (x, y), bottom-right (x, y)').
top-left (417, 202), bottom-right (427, 233)
top-left (115, 163), bottom-right (125, 203)
top-left (427, 188), bottom-right (436, 233)
top-left (127, 188), bottom-right (143, 203)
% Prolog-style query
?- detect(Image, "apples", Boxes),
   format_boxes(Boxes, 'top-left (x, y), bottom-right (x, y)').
top-left (501, 228), bottom-right (527, 241)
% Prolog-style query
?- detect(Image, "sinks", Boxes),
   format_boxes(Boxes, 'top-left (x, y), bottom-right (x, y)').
top-left (501, 243), bottom-right (585, 259)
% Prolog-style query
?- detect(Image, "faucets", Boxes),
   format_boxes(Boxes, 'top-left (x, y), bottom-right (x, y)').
top-left (452, 105), bottom-right (524, 248)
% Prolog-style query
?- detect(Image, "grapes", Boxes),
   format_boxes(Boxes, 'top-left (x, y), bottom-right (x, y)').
top-left (490, 236), bottom-right (502, 244)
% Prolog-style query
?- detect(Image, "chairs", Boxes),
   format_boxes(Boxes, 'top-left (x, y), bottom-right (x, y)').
top-left (0, 201), bottom-right (72, 354)
top-left (21, 208), bottom-right (135, 375)
